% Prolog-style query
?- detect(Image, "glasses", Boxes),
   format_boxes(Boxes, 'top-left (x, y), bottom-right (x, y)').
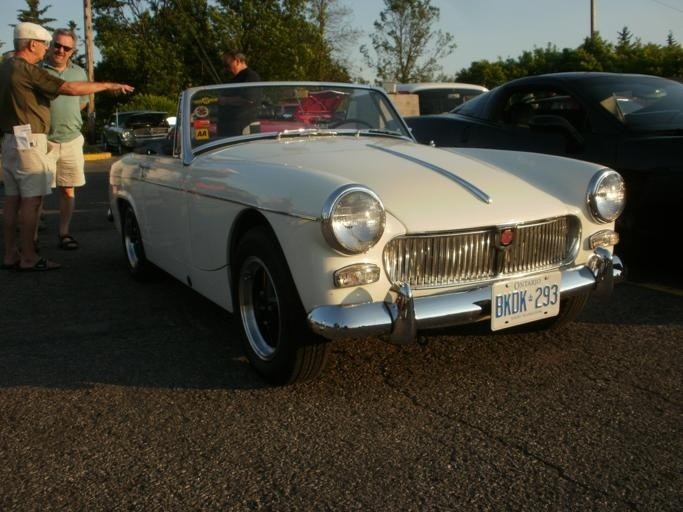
top-left (53, 40), bottom-right (73, 51)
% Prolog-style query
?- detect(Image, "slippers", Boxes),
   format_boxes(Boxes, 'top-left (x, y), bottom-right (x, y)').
top-left (2, 253), bottom-right (20, 269)
top-left (20, 257), bottom-right (61, 272)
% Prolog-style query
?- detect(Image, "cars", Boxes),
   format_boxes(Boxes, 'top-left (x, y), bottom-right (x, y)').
top-left (105, 81), bottom-right (628, 385)
top-left (389, 70), bottom-right (682, 281)
top-left (98, 109), bottom-right (174, 156)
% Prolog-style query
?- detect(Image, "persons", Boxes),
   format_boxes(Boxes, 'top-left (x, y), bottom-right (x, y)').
top-left (33, 28), bottom-right (90, 251)
top-left (216, 49), bottom-right (261, 137)
top-left (0, 21), bottom-right (135, 273)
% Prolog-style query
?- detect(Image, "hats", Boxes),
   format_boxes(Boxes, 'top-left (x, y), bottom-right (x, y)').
top-left (14, 22), bottom-right (53, 42)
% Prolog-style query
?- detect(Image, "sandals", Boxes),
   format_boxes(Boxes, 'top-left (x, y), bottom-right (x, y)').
top-left (58, 235), bottom-right (79, 250)
